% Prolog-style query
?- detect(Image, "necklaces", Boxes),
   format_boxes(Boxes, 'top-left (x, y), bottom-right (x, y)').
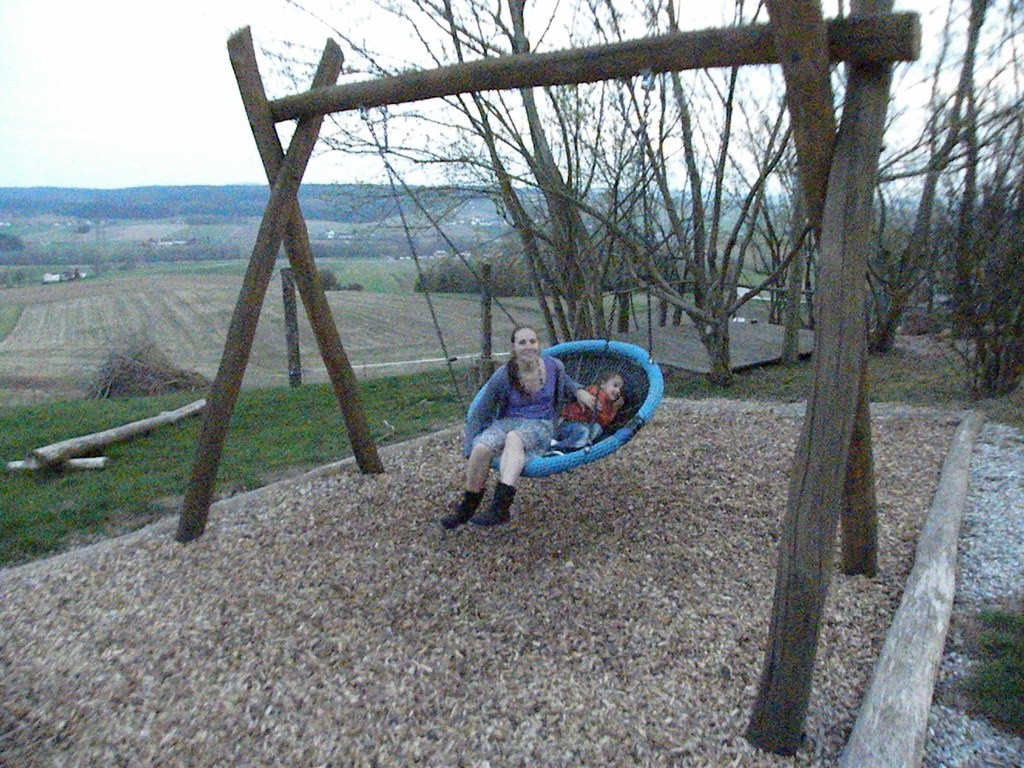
top-left (526, 361), bottom-right (544, 414)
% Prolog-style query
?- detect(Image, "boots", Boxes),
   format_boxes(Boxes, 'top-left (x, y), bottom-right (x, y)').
top-left (439, 489), bottom-right (484, 530)
top-left (468, 480), bottom-right (517, 528)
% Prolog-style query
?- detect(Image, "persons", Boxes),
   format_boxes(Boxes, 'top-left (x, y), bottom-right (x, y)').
top-left (550, 371), bottom-right (625, 456)
top-left (441, 325), bottom-right (596, 529)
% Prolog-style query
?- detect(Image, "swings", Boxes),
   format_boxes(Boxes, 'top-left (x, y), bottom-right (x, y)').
top-left (355, 67), bottom-right (666, 480)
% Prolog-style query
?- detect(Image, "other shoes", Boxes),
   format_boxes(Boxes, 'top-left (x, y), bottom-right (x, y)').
top-left (543, 450), bottom-right (564, 458)
top-left (549, 438), bottom-right (557, 451)
